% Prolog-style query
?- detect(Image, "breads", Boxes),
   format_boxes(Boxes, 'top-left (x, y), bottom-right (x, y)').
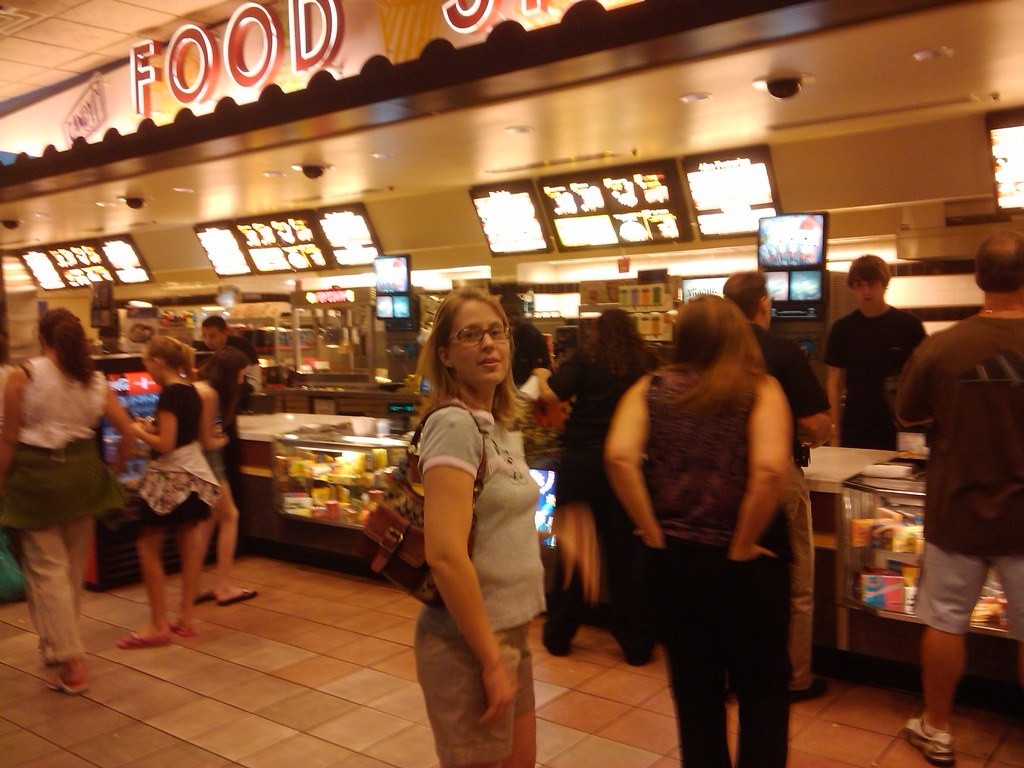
top-left (851, 508), bottom-right (1008, 631)
top-left (287, 448), bottom-right (388, 523)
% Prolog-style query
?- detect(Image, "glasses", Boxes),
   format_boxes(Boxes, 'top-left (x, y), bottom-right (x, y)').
top-left (446, 325), bottom-right (513, 351)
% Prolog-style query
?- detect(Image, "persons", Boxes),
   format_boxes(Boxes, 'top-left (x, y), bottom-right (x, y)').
top-left (498, 271), bottom-right (831, 768)
top-left (896, 232), bottom-right (1024, 763)
top-left (0, 309), bottom-right (261, 696)
top-left (823, 256), bottom-right (926, 449)
top-left (412, 285), bottom-right (548, 768)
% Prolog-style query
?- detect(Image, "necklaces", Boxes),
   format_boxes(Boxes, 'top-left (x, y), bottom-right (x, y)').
top-left (984, 307), bottom-right (1018, 313)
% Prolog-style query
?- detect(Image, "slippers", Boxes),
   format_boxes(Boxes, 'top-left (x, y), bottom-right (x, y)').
top-left (196, 590), bottom-right (215, 604)
top-left (118, 631), bottom-right (168, 649)
top-left (218, 588), bottom-right (256, 606)
top-left (168, 621), bottom-right (196, 636)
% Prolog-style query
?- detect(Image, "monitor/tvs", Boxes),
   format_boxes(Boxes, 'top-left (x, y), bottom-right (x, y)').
top-left (373, 254), bottom-right (412, 294)
top-left (758, 211), bottom-right (829, 272)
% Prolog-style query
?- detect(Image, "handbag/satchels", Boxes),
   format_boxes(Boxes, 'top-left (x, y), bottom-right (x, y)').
top-left (353, 404), bottom-right (487, 608)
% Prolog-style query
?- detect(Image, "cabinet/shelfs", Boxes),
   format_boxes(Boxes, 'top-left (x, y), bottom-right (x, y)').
top-left (843, 480), bottom-right (1014, 638)
top-left (274, 433), bottom-right (410, 530)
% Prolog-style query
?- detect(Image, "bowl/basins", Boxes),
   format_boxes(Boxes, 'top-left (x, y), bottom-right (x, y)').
top-left (349, 416), bottom-right (376, 435)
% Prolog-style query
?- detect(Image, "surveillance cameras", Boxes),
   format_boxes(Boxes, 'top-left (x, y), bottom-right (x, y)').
top-left (302, 165), bottom-right (326, 180)
top-left (126, 199), bottom-right (143, 208)
top-left (767, 78), bottom-right (802, 98)
top-left (3, 220), bottom-right (18, 228)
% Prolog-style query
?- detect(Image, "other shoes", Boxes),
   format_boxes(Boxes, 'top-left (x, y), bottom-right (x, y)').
top-left (787, 678), bottom-right (826, 702)
top-left (46, 669), bottom-right (86, 693)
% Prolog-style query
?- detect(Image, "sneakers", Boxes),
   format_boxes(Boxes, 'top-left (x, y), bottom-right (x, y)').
top-left (904, 712), bottom-right (953, 760)
